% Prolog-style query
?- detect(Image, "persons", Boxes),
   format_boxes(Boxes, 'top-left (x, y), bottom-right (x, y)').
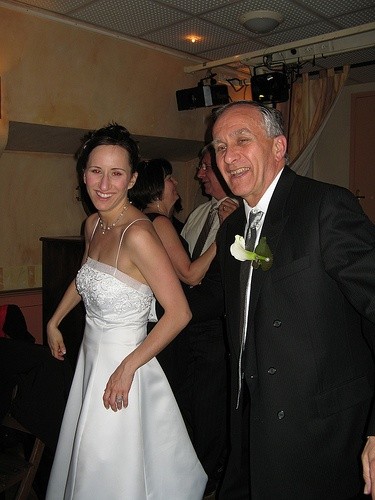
top-left (128, 144), bottom-right (231, 500)
top-left (211, 100), bottom-right (375, 500)
top-left (47, 120), bottom-right (208, 500)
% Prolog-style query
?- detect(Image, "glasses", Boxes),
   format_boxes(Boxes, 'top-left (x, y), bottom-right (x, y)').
top-left (197, 163), bottom-right (214, 170)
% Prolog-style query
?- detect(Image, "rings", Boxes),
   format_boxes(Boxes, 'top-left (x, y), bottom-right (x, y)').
top-left (116, 397), bottom-right (122, 402)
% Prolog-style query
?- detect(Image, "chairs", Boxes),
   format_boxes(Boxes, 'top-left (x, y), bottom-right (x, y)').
top-left (0, 414), bottom-right (45, 500)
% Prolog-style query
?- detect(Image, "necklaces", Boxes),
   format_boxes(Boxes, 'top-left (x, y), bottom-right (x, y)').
top-left (98, 199), bottom-right (132, 234)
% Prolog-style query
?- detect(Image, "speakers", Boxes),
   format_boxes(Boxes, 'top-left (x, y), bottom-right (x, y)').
top-left (251, 73), bottom-right (288, 103)
top-left (176, 84), bottom-right (228, 111)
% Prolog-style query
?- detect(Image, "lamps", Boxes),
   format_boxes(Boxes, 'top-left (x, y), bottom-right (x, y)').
top-left (239, 10), bottom-right (284, 33)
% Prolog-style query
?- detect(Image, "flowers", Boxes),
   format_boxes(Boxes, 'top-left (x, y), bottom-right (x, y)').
top-left (230, 234), bottom-right (273, 271)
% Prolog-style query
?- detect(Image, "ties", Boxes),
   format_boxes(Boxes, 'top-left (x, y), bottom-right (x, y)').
top-left (236, 210), bottom-right (264, 408)
top-left (191, 201), bottom-right (219, 263)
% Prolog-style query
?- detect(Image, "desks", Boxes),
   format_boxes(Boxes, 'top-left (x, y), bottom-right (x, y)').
top-left (39, 236), bottom-right (85, 363)
